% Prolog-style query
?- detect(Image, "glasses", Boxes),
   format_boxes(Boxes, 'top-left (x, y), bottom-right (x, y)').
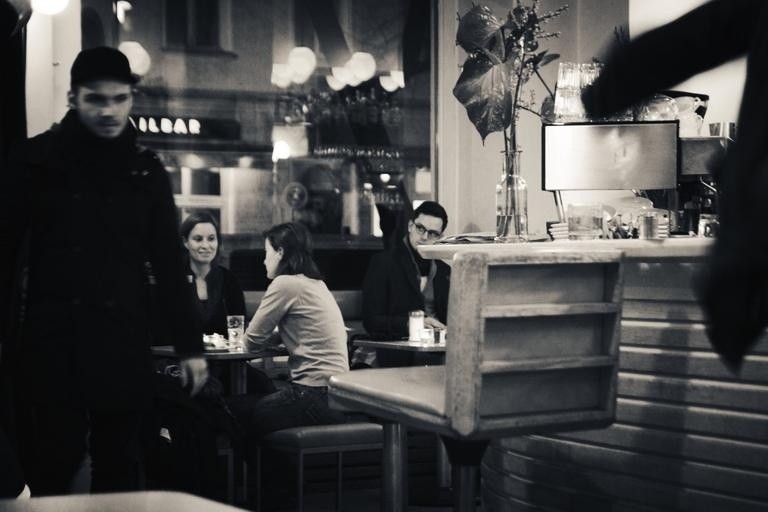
top-left (412, 221), bottom-right (439, 241)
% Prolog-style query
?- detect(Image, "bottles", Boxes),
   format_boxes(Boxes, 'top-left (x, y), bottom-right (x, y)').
top-left (408, 309), bottom-right (425, 341)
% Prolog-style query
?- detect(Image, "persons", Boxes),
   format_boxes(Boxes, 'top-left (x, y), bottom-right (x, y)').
top-left (178, 210), bottom-right (276, 500)
top-left (360, 199), bottom-right (485, 504)
top-left (2, 44), bottom-right (210, 501)
top-left (225, 223), bottom-right (353, 510)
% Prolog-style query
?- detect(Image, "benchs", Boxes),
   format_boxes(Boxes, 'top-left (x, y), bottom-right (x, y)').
top-left (229, 248), bottom-right (390, 392)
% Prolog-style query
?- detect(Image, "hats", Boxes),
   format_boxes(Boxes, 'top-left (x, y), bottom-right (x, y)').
top-left (68, 45), bottom-right (140, 88)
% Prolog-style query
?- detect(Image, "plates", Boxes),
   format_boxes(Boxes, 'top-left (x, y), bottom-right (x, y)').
top-left (550, 194), bottom-right (676, 240)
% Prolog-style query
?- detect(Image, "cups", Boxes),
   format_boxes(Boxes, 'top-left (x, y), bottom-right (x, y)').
top-left (419, 328), bottom-right (434, 343)
top-left (565, 203), bottom-right (602, 239)
top-left (636, 213), bottom-right (655, 240)
top-left (226, 314), bottom-right (245, 347)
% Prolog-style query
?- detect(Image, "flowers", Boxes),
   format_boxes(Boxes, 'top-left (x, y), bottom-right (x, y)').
top-left (451, 0), bottom-right (571, 238)
